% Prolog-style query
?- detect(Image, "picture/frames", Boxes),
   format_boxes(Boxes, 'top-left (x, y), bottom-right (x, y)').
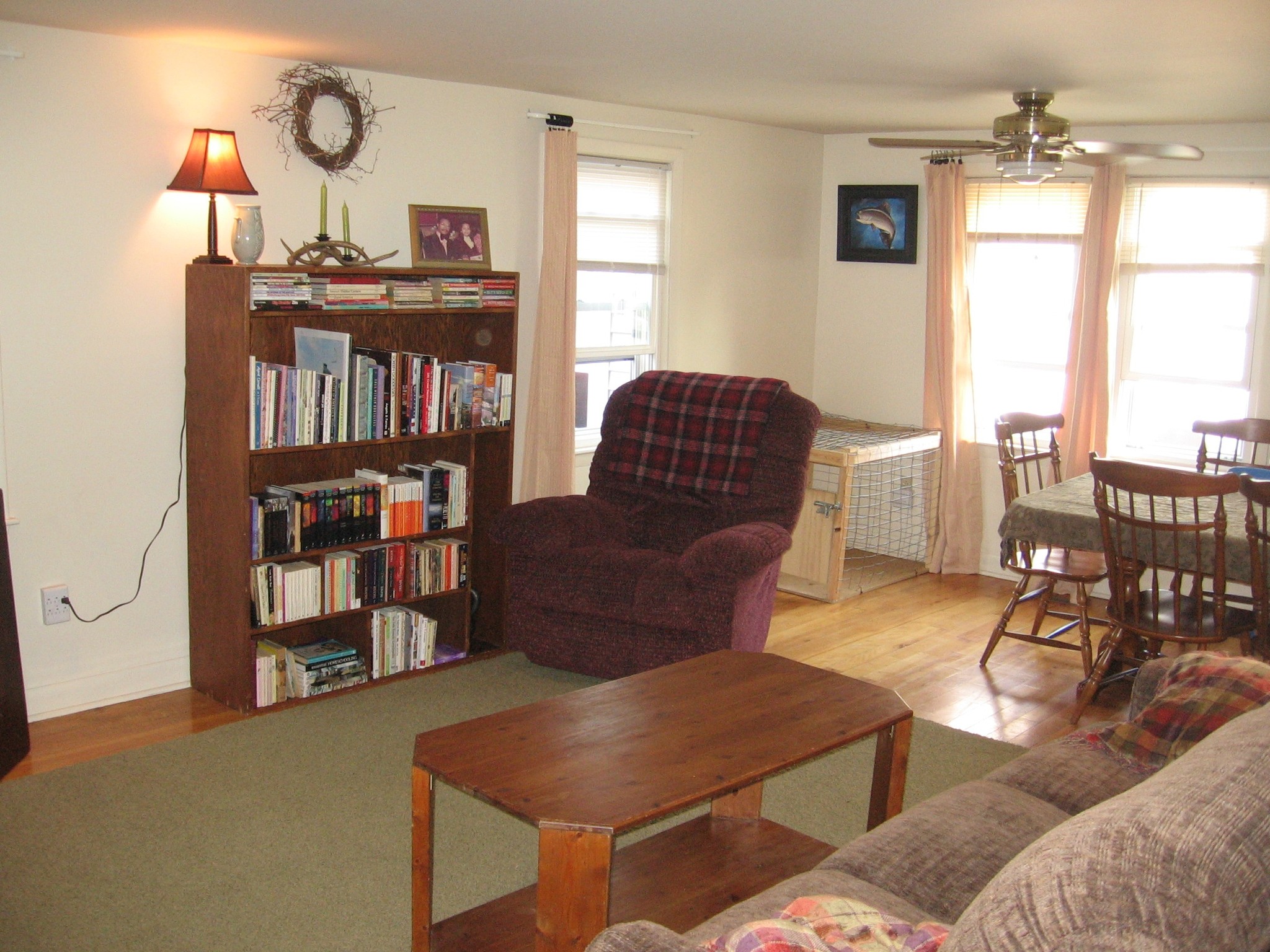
top-left (836, 183), bottom-right (922, 265)
top-left (408, 202), bottom-right (494, 270)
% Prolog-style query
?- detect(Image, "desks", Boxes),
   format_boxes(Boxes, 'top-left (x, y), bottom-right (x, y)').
top-left (997, 465), bottom-right (1270, 603)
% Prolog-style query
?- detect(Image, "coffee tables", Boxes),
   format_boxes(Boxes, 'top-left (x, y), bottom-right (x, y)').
top-left (410, 649), bottom-right (915, 952)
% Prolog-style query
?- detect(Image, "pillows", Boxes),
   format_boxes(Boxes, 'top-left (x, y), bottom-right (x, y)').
top-left (705, 891), bottom-right (950, 952)
top-left (1074, 650), bottom-right (1270, 775)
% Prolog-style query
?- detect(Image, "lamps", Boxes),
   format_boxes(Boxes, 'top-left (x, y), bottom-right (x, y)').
top-left (167, 128), bottom-right (254, 263)
top-left (995, 151), bottom-right (1065, 184)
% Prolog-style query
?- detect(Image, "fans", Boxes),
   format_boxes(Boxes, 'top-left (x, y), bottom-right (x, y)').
top-left (869, 91), bottom-right (1204, 163)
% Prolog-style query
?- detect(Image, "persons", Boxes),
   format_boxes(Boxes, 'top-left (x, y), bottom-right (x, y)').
top-left (422, 217), bottom-right (482, 261)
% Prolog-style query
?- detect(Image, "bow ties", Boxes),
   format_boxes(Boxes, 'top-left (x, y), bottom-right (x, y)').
top-left (441, 234), bottom-right (449, 240)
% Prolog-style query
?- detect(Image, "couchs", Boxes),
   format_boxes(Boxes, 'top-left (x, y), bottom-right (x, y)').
top-left (506, 369), bottom-right (813, 672)
top-left (591, 641), bottom-right (1270, 952)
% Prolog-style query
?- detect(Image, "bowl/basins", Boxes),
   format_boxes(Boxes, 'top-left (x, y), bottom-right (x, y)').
top-left (1227, 466), bottom-right (1270, 482)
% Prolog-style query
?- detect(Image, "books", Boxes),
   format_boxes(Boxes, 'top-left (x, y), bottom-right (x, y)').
top-left (249, 461), bottom-right (469, 561)
top-left (256, 606), bottom-right (438, 708)
top-left (249, 273), bottom-right (516, 311)
top-left (251, 538), bottom-right (470, 628)
top-left (250, 327), bottom-right (513, 451)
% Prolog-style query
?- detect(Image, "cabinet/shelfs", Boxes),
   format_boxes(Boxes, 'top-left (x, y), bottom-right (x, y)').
top-left (184, 261), bottom-right (524, 711)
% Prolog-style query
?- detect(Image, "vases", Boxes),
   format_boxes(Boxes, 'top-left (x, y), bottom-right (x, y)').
top-left (231, 204), bottom-right (265, 265)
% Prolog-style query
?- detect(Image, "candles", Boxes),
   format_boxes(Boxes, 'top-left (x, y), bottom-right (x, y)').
top-left (342, 201), bottom-right (352, 257)
top-left (320, 179), bottom-right (328, 233)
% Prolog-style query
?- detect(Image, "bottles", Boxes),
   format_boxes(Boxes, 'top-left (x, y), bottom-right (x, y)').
top-left (232, 204), bottom-right (264, 265)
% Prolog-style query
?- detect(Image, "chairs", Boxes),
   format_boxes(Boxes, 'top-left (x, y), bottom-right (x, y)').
top-left (1235, 471), bottom-right (1270, 664)
top-left (1072, 451), bottom-right (1240, 727)
top-left (979, 407), bottom-right (1146, 665)
top-left (1192, 415), bottom-right (1270, 492)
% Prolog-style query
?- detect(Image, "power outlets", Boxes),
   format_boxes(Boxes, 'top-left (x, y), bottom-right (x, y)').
top-left (42, 584), bottom-right (75, 622)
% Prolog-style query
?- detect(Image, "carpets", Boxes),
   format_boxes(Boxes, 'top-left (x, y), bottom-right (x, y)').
top-left (0, 644), bottom-right (1027, 952)
